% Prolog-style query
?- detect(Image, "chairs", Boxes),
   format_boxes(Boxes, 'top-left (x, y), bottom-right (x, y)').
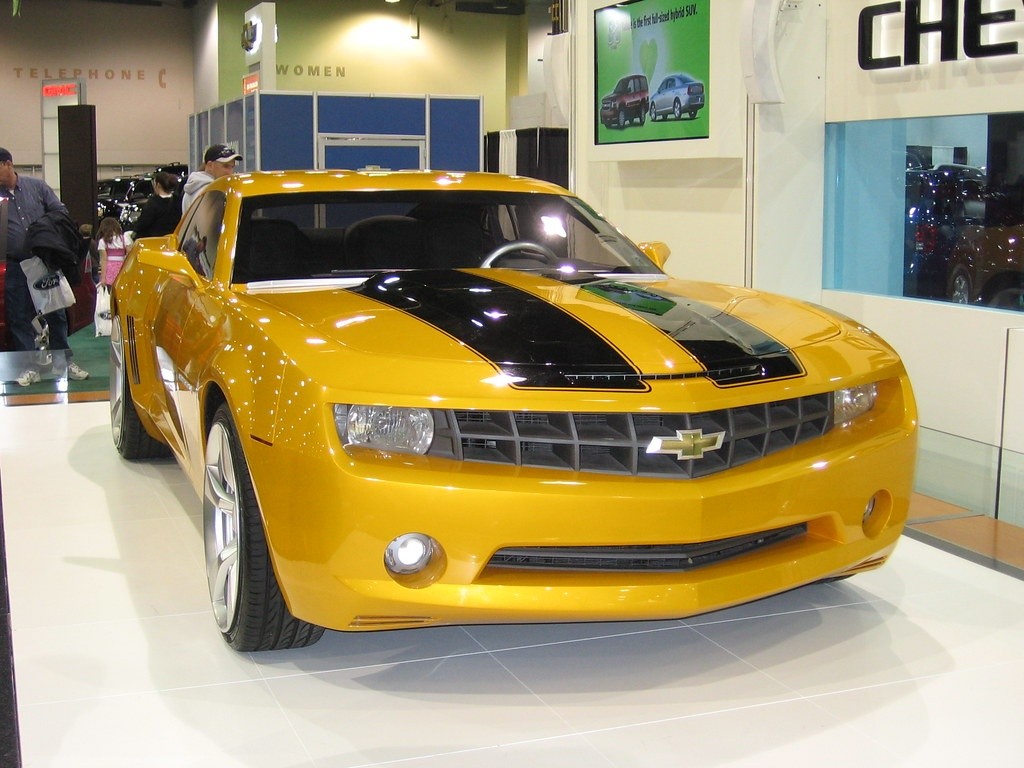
top-left (233, 217), bottom-right (315, 284)
top-left (421, 215), bottom-right (484, 265)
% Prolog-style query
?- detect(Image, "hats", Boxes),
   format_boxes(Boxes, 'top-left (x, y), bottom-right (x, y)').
top-left (204, 144), bottom-right (243, 163)
top-left (0, 147), bottom-right (12, 161)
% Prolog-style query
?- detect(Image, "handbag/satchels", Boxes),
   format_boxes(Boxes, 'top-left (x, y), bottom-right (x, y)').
top-left (95, 282), bottom-right (113, 338)
top-left (19, 255), bottom-right (77, 314)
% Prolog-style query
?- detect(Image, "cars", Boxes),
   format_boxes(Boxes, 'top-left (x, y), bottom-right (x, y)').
top-left (647, 72), bottom-right (705, 123)
top-left (900, 147), bottom-right (1024, 309)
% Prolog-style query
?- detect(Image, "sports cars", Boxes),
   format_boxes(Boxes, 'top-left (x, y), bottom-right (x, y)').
top-left (109, 166), bottom-right (921, 657)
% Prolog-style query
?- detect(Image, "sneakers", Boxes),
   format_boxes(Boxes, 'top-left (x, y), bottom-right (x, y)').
top-left (14, 367), bottom-right (41, 386)
top-left (52, 363), bottom-right (89, 380)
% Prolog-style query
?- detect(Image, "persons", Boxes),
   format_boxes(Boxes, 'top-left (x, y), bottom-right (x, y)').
top-left (0, 147), bottom-right (90, 387)
top-left (182, 144), bottom-right (243, 215)
top-left (184, 237), bottom-right (208, 277)
top-left (79, 171), bottom-right (184, 295)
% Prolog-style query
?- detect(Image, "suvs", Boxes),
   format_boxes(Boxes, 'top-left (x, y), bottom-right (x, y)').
top-left (599, 75), bottom-right (651, 130)
